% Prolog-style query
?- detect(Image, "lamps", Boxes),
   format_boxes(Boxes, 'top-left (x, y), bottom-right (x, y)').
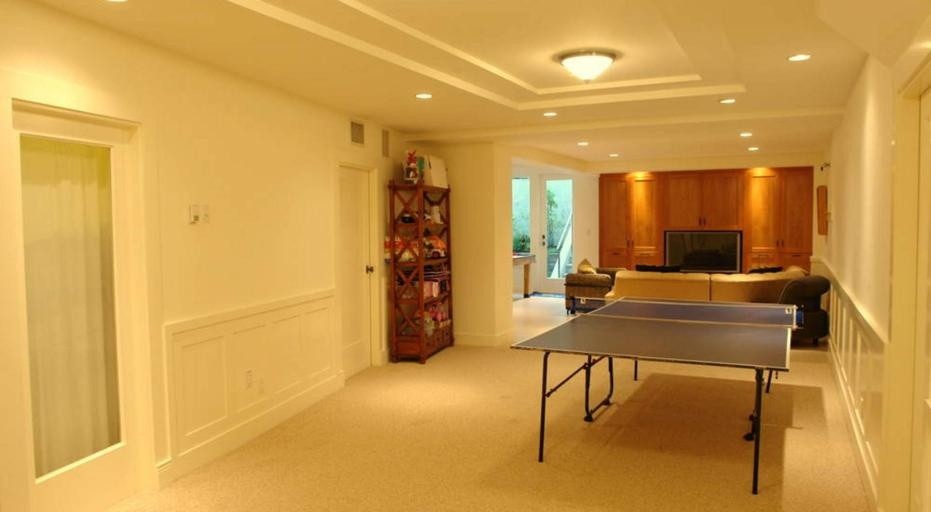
top-left (554, 49), bottom-right (617, 86)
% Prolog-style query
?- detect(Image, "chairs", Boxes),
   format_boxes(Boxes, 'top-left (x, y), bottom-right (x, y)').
top-left (564, 267), bottom-right (626, 315)
top-left (774, 275), bottom-right (829, 346)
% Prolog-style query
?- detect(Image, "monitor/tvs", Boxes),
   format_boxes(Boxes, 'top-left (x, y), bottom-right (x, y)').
top-left (665, 230), bottom-right (741, 273)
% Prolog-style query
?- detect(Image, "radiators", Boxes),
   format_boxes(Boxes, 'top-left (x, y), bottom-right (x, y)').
top-left (829, 270), bottom-right (885, 506)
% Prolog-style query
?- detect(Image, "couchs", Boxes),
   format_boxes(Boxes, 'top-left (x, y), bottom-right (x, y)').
top-left (603, 265), bottom-right (808, 312)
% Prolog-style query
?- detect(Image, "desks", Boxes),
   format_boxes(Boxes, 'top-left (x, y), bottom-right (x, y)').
top-left (512, 251), bottom-right (536, 299)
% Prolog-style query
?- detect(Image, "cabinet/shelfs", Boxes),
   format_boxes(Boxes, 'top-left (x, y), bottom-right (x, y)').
top-left (598, 165), bottom-right (814, 272)
top-left (385, 176), bottom-right (453, 364)
